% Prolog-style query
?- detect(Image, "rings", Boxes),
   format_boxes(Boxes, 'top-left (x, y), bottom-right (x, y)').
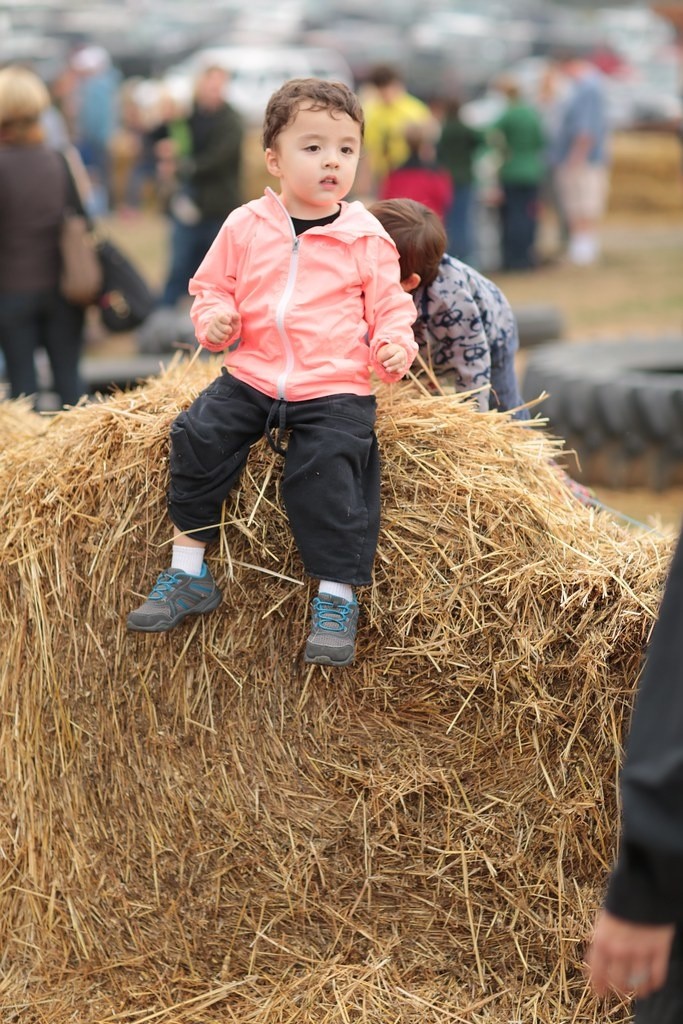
top-left (630, 976), bottom-right (647, 984)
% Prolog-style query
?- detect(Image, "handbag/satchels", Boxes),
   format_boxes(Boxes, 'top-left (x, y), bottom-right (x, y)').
top-left (59, 208), bottom-right (152, 329)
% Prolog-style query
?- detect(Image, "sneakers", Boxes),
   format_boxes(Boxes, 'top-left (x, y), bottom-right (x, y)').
top-left (125, 562), bottom-right (222, 632)
top-left (303, 591), bottom-right (360, 666)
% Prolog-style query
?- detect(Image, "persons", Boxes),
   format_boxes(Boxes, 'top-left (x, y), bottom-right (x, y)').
top-left (586, 534), bottom-right (683, 1024)
top-left (126, 78), bottom-right (418, 664)
top-left (369, 198), bottom-right (597, 505)
top-left (541, 45), bottom-right (611, 264)
top-left (0, 45), bottom-right (550, 411)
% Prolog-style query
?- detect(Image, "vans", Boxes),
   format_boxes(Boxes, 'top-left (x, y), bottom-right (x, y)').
top-left (142, 47), bottom-right (352, 121)
